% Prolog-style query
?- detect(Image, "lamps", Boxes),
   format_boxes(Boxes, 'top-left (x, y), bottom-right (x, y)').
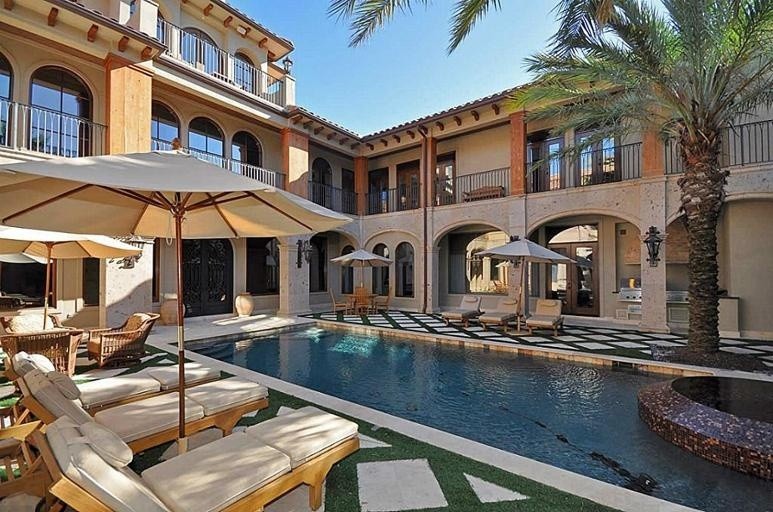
top-left (295, 238), bottom-right (313, 268)
top-left (641, 225), bottom-right (668, 267)
top-left (122, 235), bottom-right (145, 269)
top-left (283, 56), bottom-right (293, 74)
top-left (508, 235), bottom-right (523, 270)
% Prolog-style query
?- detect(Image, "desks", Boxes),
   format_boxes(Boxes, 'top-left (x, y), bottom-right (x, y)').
top-left (344, 292), bottom-right (378, 314)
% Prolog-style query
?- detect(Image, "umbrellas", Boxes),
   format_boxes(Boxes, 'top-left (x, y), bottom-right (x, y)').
top-left (329, 249), bottom-right (394, 287)
top-left (474, 238), bottom-right (578, 314)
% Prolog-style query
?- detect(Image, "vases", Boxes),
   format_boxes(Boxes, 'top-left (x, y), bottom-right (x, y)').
top-left (160, 299), bottom-right (186, 325)
top-left (235, 292), bottom-right (255, 316)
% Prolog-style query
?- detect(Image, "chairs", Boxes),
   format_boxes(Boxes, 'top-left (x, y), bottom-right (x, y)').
top-left (375, 286), bottom-right (394, 314)
top-left (1, 366), bottom-right (269, 506)
top-left (0, 329), bottom-right (84, 378)
top-left (329, 287), bottom-right (348, 315)
top-left (87, 312), bottom-right (159, 368)
top-left (356, 286), bottom-right (369, 314)
top-left (441, 293), bottom-right (486, 328)
top-left (31, 405), bottom-right (362, 510)
top-left (0, 350), bottom-right (224, 427)
top-left (526, 298), bottom-right (566, 337)
top-left (480, 295), bottom-right (523, 334)
top-left (1, 313), bottom-right (66, 332)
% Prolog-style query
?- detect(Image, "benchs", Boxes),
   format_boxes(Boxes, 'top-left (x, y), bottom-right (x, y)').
top-left (461, 185), bottom-right (504, 202)
